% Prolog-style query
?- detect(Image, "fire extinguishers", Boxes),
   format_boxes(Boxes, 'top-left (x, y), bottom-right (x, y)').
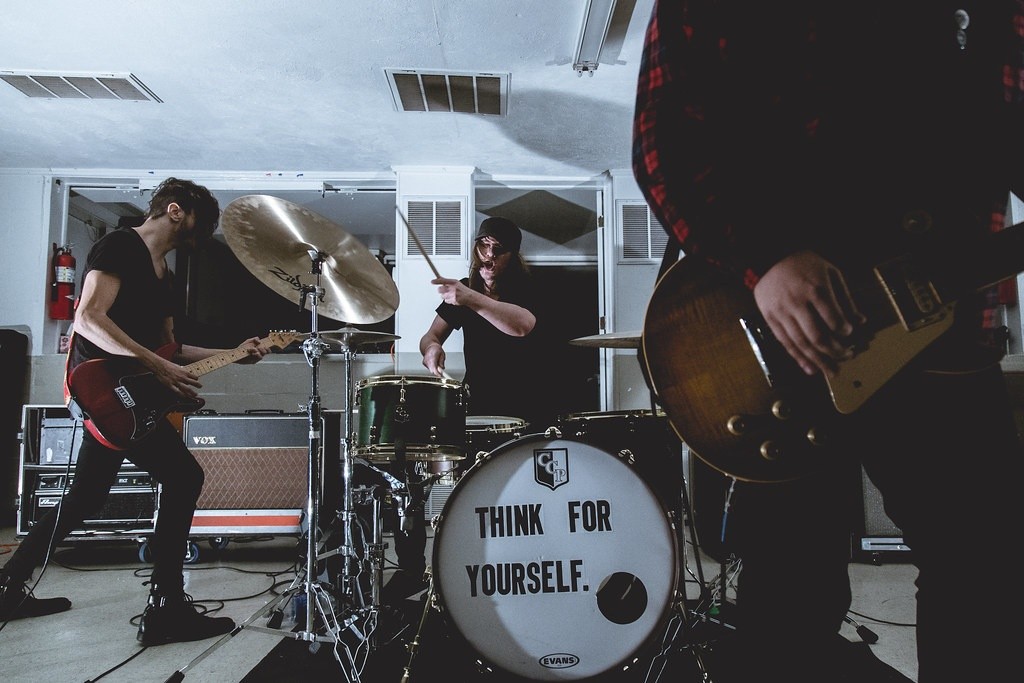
top-left (49, 239), bottom-right (77, 322)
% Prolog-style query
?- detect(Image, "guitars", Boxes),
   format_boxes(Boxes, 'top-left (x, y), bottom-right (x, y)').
top-left (66, 330), bottom-right (303, 450)
top-left (643, 220), bottom-right (1024, 484)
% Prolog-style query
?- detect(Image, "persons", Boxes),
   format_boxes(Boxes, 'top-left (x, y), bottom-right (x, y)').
top-left (632, 0), bottom-right (1024, 683)
top-left (0, 179), bottom-right (273, 645)
top-left (420, 217), bottom-right (555, 417)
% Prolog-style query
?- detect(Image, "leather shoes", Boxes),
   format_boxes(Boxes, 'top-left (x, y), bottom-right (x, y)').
top-left (136, 593), bottom-right (236, 645)
top-left (0, 569), bottom-right (72, 623)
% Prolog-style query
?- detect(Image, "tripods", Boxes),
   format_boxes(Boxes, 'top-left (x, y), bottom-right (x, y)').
top-left (166, 259), bottom-right (442, 683)
top-left (644, 559), bottom-right (879, 683)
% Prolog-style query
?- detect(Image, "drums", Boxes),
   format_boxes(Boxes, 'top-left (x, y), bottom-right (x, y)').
top-left (431, 433), bottom-right (680, 683)
top-left (567, 409), bottom-right (687, 527)
top-left (429, 416), bottom-right (528, 486)
top-left (349, 375), bottom-right (467, 460)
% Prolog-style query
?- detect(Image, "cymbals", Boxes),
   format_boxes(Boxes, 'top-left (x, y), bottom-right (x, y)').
top-left (221, 195), bottom-right (401, 325)
top-left (294, 328), bottom-right (402, 346)
top-left (569, 331), bottom-right (643, 349)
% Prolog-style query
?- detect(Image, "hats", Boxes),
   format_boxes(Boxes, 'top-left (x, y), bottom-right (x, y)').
top-left (476, 216), bottom-right (522, 256)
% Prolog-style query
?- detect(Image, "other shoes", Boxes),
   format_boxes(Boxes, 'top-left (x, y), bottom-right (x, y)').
top-left (382, 563), bottom-right (430, 604)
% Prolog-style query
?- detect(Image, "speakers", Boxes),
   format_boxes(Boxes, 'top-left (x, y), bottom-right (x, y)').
top-left (838, 464), bottom-right (903, 537)
top-left (184, 412), bottom-right (342, 536)
